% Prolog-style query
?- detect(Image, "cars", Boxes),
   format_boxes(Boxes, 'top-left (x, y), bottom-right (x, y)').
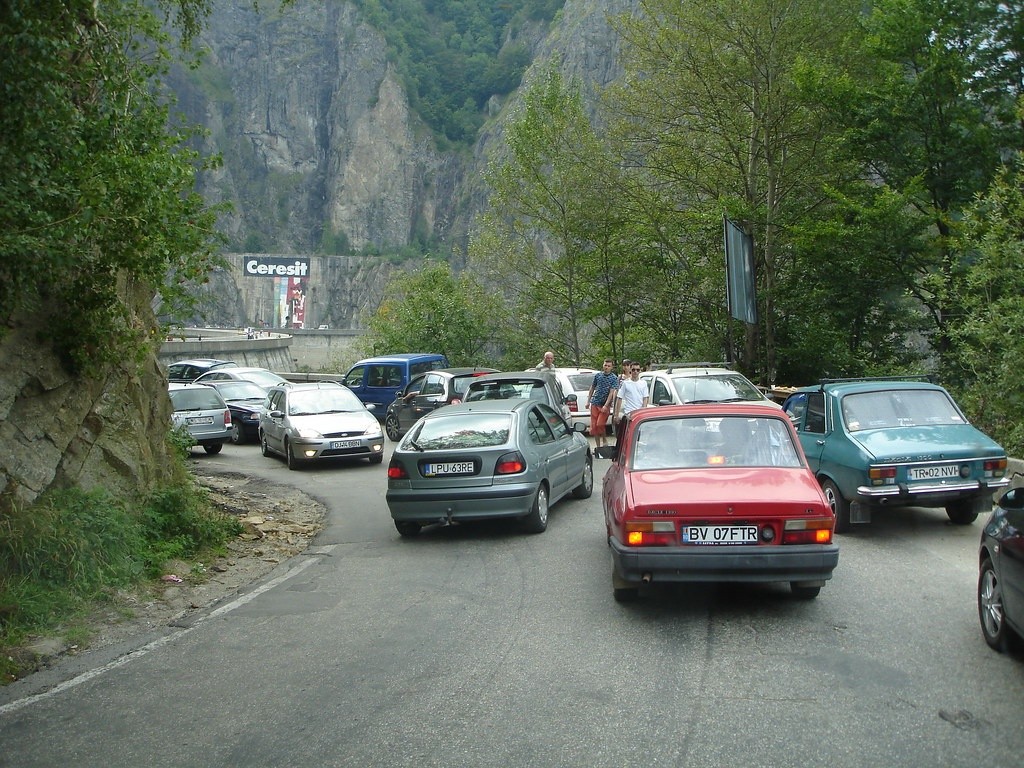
top-left (462, 372), bottom-right (578, 437)
top-left (385, 399), bottom-right (594, 536)
top-left (781, 376), bottom-right (1010, 535)
top-left (977, 487), bottom-right (1024, 651)
top-left (258, 380), bottom-right (384, 470)
top-left (189, 367), bottom-right (296, 393)
top-left (385, 367), bottom-right (504, 442)
top-left (594, 406), bottom-right (840, 603)
top-left (192, 380), bottom-right (269, 445)
top-left (512, 367), bottom-right (618, 434)
top-left (638, 363), bottom-right (795, 433)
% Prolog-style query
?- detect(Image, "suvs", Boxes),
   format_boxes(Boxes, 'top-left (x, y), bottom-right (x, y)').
top-left (168, 359), bottom-right (240, 384)
top-left (168, 383), bottom-right (235, 455)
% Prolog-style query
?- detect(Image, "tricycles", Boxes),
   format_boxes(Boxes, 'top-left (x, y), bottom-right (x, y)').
top-left (247, 332), bottom-right (254, 339)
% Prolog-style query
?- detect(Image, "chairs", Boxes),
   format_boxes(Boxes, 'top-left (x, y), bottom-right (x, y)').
top-left (652, 426), bottom-right (679, 453)
top-left (719, 418), bottom-right (751, 442)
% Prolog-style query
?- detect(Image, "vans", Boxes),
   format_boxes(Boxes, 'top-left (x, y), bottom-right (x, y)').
top-left (338, 353), bottom-right (448, 419)
top-left (318, 325), bottom-right (328, 330)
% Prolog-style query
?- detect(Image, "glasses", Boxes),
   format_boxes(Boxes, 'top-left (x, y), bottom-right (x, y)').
top-left (631, 368), bottom-right (641, 373)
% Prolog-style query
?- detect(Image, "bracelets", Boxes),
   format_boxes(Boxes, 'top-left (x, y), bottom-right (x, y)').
top-left (614, 417), bottom-right (617, 419)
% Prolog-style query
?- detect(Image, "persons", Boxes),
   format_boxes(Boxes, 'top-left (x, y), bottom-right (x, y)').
top-left (613, 359), bottom-right (632, 425)
top-left (613, 361), bottom-right (653, 439)
top-left (403, 390), bottom-right (421, 400)
top-left (535, 351), bottom-right (556, 379)
top-left (585, 358), bottom-right (620, 447)
